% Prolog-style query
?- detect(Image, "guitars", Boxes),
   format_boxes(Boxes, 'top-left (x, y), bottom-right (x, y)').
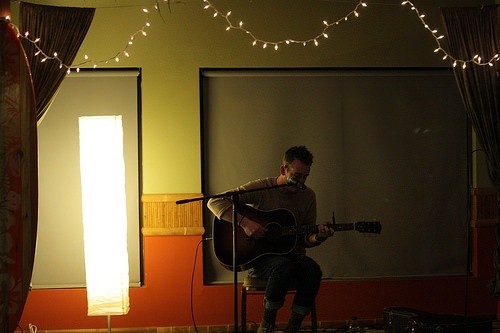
top-left (210, 203), bottom-right (383, 272)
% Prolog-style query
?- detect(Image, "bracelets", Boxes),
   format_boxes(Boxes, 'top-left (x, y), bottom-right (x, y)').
top-left (238, 215), bottom-right (244, 226)
top-left (314, 235), bottom-right (327, 242)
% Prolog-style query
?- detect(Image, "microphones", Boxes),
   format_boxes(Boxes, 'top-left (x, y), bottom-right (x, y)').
top-left (287, 175), bottom-right (307, 191)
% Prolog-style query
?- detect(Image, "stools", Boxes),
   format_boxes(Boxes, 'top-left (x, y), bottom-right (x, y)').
top-left (240, 261), bottom-right (318, 333)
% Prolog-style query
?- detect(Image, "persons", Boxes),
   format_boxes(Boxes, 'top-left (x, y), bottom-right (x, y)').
top-left (207, 146), bottom-right (337, 333)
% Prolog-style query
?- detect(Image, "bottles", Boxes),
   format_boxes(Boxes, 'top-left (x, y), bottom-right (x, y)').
top-left (349, 315), bottom-right (360, 333)
